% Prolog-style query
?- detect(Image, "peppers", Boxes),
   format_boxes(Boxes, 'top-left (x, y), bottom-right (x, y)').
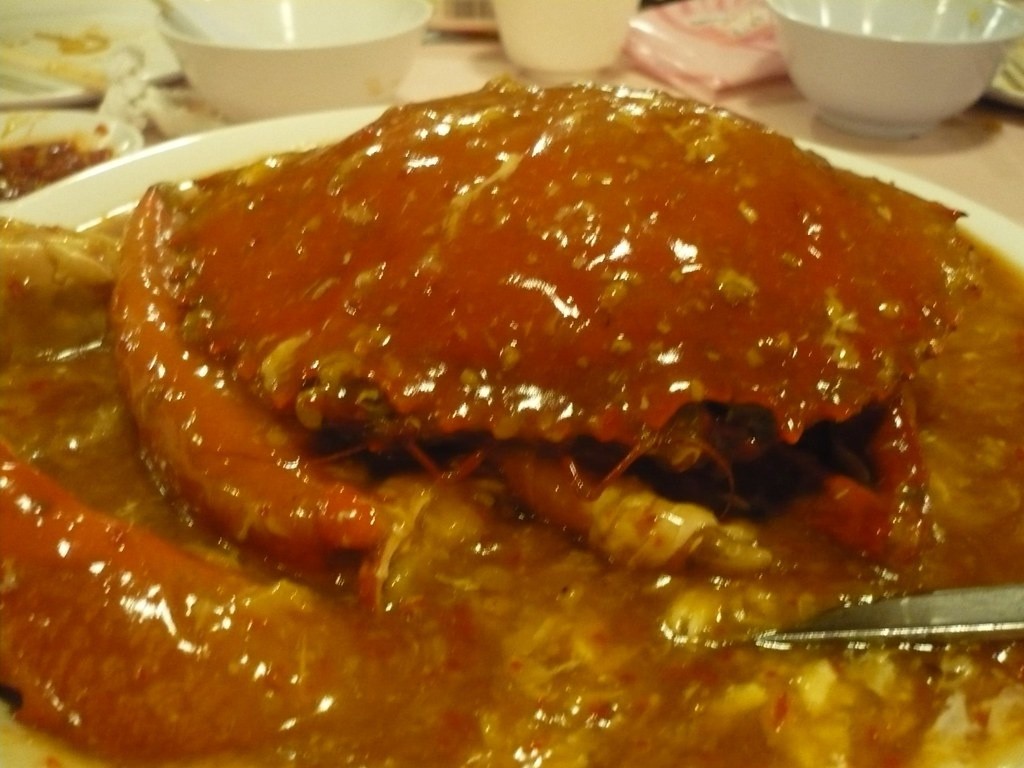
top-left (115, 182), bottom-right (395, 596)
top-left (0, 432), bottom-right (370, 768)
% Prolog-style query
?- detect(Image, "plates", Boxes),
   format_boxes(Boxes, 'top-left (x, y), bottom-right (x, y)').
top-left (0, 110), bottom-right (146, 197)
top-left (0, 0), bottom-right (183, 107)
top-left (0, 106), bottom-right (1024, 307)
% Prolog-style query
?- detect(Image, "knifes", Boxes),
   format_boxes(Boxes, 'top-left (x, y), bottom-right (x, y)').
top-left (760, 582), bottom-right (1024, 641)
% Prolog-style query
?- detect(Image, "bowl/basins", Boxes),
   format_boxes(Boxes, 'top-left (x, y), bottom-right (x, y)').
top-left (491, 1), bottom-right (638, 74)
top-left (760, 0), bottom-right (1024, 140)
top-left (158, 1), bottom-right (433, 121)
